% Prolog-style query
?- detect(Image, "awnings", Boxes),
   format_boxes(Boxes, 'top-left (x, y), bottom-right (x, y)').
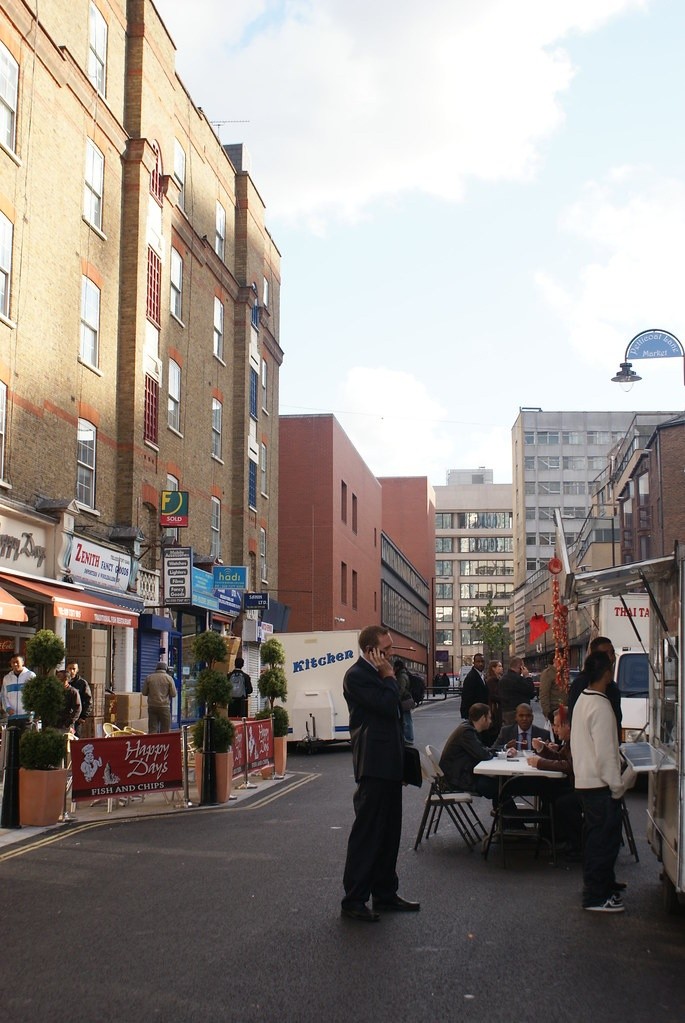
top-left (577, 598), bottom-right (599, 630)
top-left (564, 553), bottom-right (678, 682)
top-left (0, 574), bottom-right (140, 628)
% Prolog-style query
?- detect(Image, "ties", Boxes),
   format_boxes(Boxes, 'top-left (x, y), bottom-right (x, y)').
top-left (521, 732), bottom-right (528, 749)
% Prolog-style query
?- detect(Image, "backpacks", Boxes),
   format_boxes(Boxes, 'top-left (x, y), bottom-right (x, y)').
top-left (399, 667), bottom-right (426, 702)
top-left (227, 672), bottom-right (247, 700)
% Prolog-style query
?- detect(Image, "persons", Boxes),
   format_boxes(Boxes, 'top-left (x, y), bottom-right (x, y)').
top-left (531, 707), bottom-right (582, 856)
top-left (440, 703), bottom-right (527, 830)
top-left (492, 703), bottom-right (565, 795)
top-left (569, 637), bottom-right (625, 910)
top-left (340, 626), bottom-right (423, 921)
top-left (144, 661), bottom-right (177, 734)
top-left (1, 654), bottom-right (93, 738)
top-left (394, 660), bottom-right (426, 745)
top-left (436, 673), bottom-right (450, 694)
top-left (461, 654), bottom-right (567, 747)
top-left (227, 658), bottom-right (253, 717)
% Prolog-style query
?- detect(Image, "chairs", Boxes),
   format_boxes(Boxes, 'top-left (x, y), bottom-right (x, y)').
top-left (413, 743), bottom-right (488, 851)
top-left (172, 736), bottom-right (196, 802)
top-left (485, 775), bottom-right (557, 871)
top-left (90, 721), bottom-right (148, 813)
top-left (579, 750), bottom-right (641, 863)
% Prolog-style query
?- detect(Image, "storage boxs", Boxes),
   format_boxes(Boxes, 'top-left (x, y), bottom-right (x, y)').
top-left (66, 628), bottom-right (148, 737)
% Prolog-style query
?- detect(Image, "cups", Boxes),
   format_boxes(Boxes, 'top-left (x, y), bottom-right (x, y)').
top-left (496, 752), bottom-right (508, 759)
top-left (523, 749), bottom-right (535, 760)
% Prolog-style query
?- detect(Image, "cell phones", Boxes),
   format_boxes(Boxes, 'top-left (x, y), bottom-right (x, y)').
top-left (367, 648), bottom-right (386, 657)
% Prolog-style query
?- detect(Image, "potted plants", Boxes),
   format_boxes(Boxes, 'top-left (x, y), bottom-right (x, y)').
top-left (258, 637), bottom-right (289, 777)
top-left (18, 628), bottom-right (69, 827)
top-left (190, 630), bottom-right (236, 803)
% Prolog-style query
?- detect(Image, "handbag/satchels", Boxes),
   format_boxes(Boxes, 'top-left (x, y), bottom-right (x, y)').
top-left (400, 697), bottom-right (416, 711)
top-left (402, 748), bottom-right (422, 788)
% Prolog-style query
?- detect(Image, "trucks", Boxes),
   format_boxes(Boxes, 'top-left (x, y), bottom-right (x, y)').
top-left (456, 666), bottom-right (472, 688)
top-left (598, 593), bottom-right (651, 744)
top-left (266, 629), bottom-right (363, 741)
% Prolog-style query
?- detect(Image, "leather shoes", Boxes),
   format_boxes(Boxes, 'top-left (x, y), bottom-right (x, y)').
top-left (372, 893), bottom-right (421, 911)
top-left (341, 904), bottom-right (381, 921)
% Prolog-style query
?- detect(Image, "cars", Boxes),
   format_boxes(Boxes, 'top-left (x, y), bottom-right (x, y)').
top-left (528, 669), bottom-right (581, 696)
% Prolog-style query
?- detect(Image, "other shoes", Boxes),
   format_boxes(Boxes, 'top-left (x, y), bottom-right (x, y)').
top-left (610, 881), bottom-right (627, 889)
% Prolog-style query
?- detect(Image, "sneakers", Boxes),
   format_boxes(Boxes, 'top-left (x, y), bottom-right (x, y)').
top-left (583, 899), bottom-right (626, 912)
top-left (609, 894), bottom-right (624, 906)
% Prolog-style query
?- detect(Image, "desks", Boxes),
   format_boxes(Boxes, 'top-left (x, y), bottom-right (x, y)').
top-left (474, 756), bottom-right (569, 855)
